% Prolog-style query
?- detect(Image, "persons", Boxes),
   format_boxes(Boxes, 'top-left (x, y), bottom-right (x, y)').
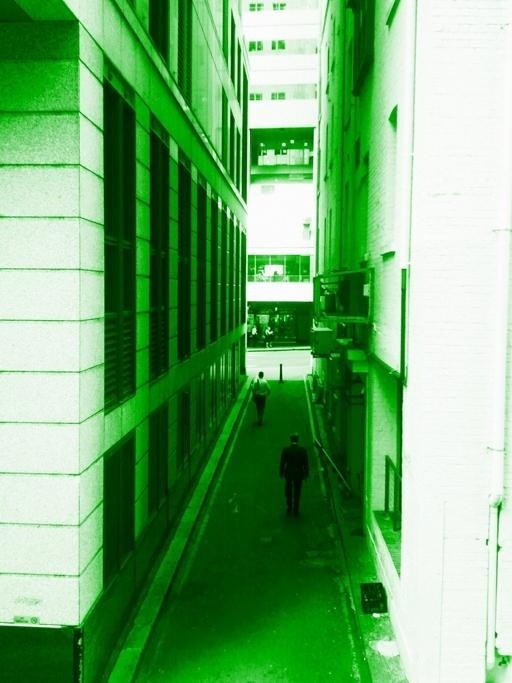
top-left (252, 326), bottom-right (258, 343)
top-left (280, 431), bottom-right (308, 516)
top-left (264, 327), bottom-right (273, 348)
top-left (252, 371), bottom-right (271, 423)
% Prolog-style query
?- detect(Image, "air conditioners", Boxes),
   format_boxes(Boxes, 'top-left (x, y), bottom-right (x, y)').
top-left (308, 326), bottom-right (333, 354)
